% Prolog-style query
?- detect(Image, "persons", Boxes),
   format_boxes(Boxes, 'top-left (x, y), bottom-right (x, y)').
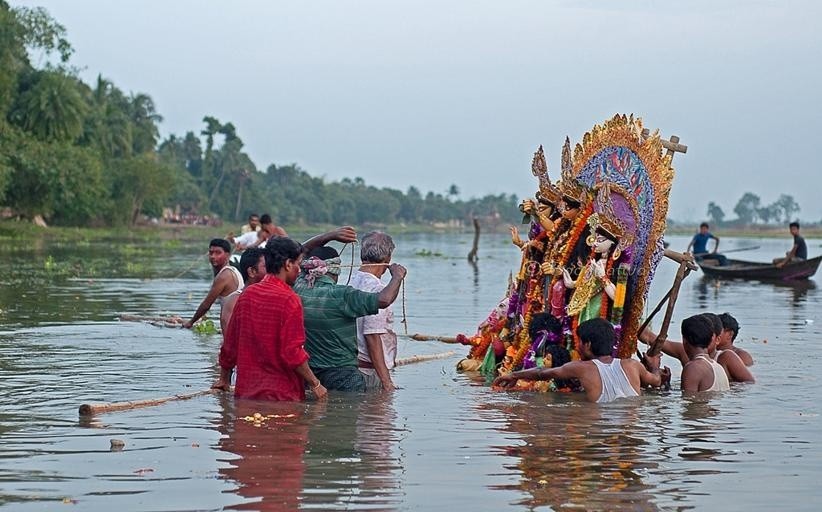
top-left (715, 312), bottom-right (754, 368)
top-left (179, 213), bottom-right (407, 402)
top-left (453, 180), bottom-right (635, 398)
top-left (773, 222), bottom-right (807, 268)
top-left (687, 223), bottom-right (727, 265)
top-left (662, 314), bottom-right (730, 397)
top-left (634, 312), bottom-right (757, 388)
top-left (491, 317), bottom-right (663, 404)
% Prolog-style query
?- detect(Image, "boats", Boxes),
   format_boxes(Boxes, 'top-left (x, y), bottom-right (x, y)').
top-left (685, 250), bottom-right (822, 283)
